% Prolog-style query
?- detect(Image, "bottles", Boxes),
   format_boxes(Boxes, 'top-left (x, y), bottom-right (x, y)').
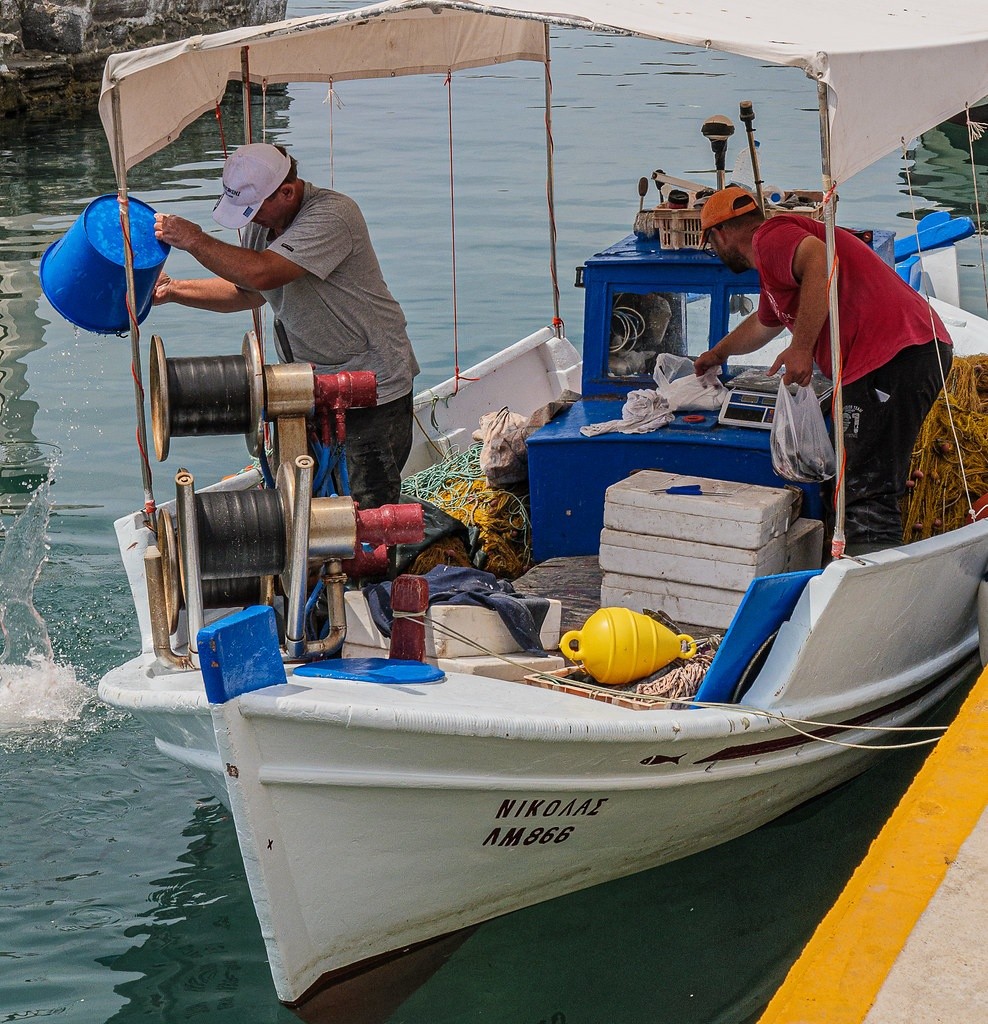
top-left (731, 140), bottom-right (760, 191)
top-left (760, 183), bottom-right (786, 204)
top-left (668, 189), bottom-right (690, 209)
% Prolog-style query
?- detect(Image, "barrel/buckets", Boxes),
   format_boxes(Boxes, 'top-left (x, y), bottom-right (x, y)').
top-left (40, 194), bottom-right (171, 334)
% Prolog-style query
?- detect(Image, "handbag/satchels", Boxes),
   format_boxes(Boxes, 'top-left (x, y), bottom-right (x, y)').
top-left (653, 352), bottom-right (730, 412)
top-left (770, 375), bottom-right (835, 485)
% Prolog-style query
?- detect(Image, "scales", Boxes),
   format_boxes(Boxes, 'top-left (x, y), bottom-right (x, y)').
top-left (717, 367), bottom-right (834, 430)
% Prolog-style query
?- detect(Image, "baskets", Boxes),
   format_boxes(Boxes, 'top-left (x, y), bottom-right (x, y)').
top-left (654, 195), bottom-right (712, 250)
top-left (763, 189), bottom-right (827, 221)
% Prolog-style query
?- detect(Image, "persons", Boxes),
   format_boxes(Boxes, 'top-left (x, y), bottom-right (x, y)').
top-left (694, 184), bottom-right (954, 529)
top-left (150, 142), bottom-right (421, 510)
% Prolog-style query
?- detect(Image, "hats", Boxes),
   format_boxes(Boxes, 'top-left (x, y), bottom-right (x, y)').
top-left (211, 143), bottom-right (291, 229)
top-left (696, 186), bottom-right (758, 251)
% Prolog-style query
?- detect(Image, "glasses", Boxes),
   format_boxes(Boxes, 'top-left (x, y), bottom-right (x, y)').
top-left (702, 230), bottom-right (719, 257)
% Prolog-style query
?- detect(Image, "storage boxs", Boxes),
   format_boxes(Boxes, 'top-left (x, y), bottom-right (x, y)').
top-left (602, 470), bottom-right (793, 548)
top-left (598, 528), bottom-right (788, 592)
top-left (655, 187), bottom-right (842, 254)
top-left (786, 517), bottom-right (825, 569)
top-left (598, 570), bottom-right (746, 629)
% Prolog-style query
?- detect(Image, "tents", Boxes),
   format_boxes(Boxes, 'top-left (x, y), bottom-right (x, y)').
top-left (98, 3), bottom-right (987, 191)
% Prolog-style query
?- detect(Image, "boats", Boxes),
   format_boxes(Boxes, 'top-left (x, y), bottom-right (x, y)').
top-left (94, 5), bottom-right (988, 1002)
